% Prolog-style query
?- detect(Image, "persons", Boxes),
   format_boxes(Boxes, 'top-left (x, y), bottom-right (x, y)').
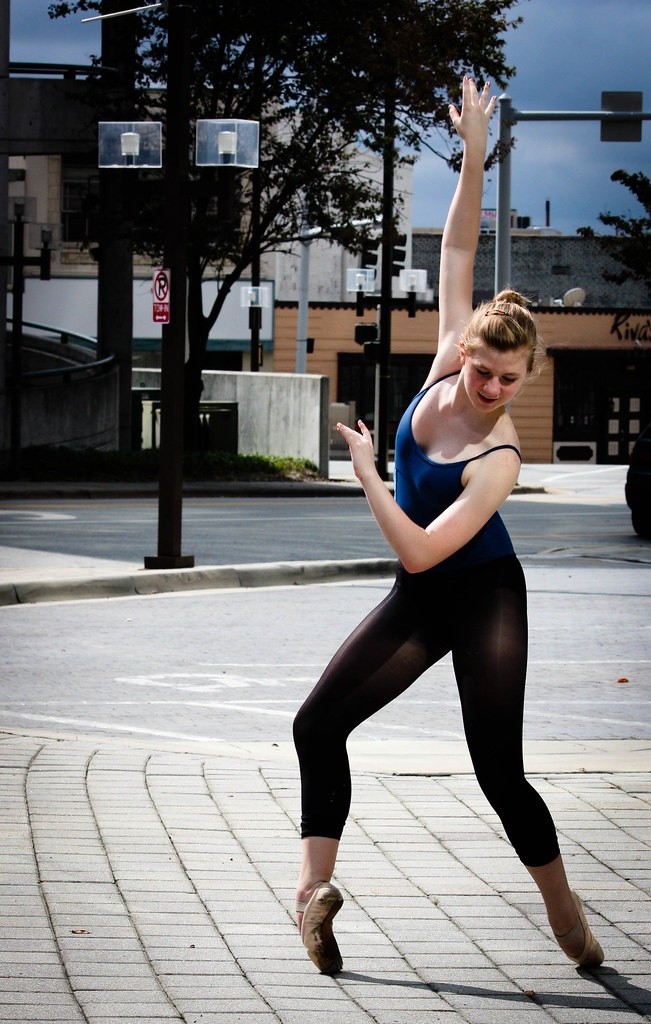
top-left (286, 70), bottom-right (605, 981)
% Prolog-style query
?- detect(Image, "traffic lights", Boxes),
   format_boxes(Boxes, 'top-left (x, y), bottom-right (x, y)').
top-left (353, 323), bottom-right (379, 347)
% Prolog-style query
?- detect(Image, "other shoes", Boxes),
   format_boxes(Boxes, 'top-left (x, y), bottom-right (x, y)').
top-left (296, 882), bottom-right (344, 975)
top-left (564, 886), bottom-right (605, 971)
